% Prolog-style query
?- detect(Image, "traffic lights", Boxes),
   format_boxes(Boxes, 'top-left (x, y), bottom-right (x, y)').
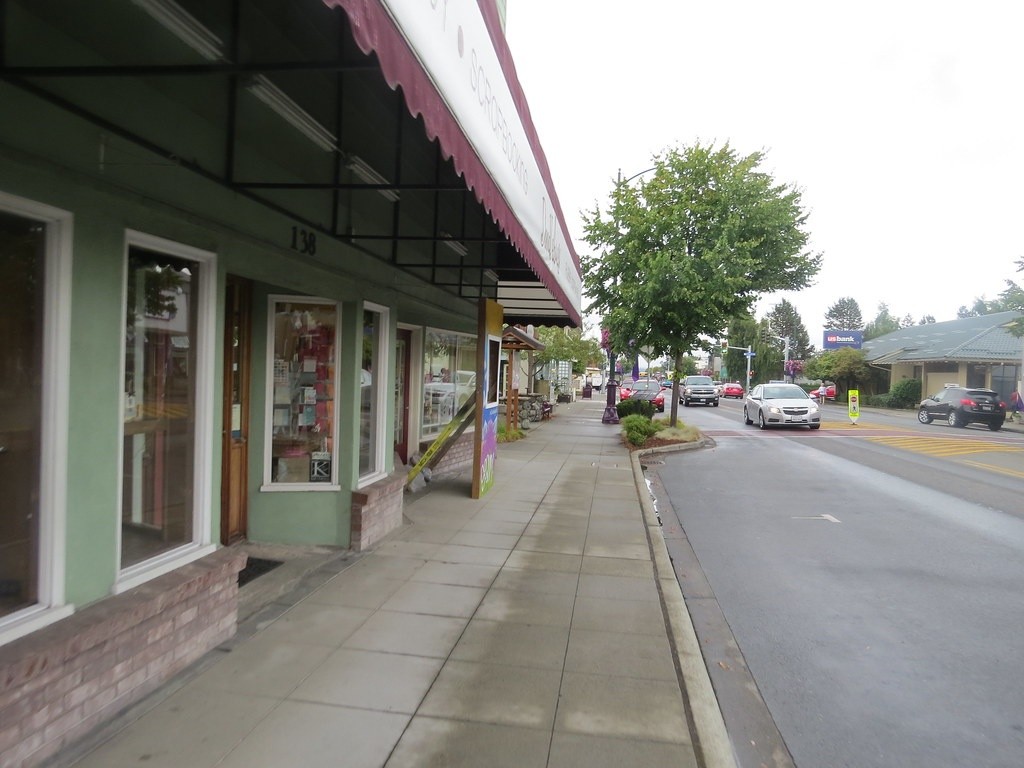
top-left (721, 342), bottom-right (728, 355)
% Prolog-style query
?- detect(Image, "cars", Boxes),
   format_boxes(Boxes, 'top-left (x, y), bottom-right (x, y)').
top-left (713, 381), bottom-right (744, 399)
top-left (743, 383), bottom-right (821, 430)
top-left (809, 385), bottom-right (835, 399)
top-left (618, 376), bottom-right (672, 411)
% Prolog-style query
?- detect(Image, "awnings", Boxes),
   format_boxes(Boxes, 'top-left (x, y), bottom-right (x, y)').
top-left (0, 0), bottom-right (582, 330)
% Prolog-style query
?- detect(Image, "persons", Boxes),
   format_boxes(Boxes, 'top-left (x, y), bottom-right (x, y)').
top-left (818, 383), bottom-right (826, 404)
top-left (1010, 388), bottom-right (1019, 417)
top-left (426, 368), bottom-right (449, 414)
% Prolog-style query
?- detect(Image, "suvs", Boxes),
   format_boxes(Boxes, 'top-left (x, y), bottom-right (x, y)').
top-left (678, 375), bottom-right (720, 407)
top-left (917, 386), bottom-right (1007, 431)
